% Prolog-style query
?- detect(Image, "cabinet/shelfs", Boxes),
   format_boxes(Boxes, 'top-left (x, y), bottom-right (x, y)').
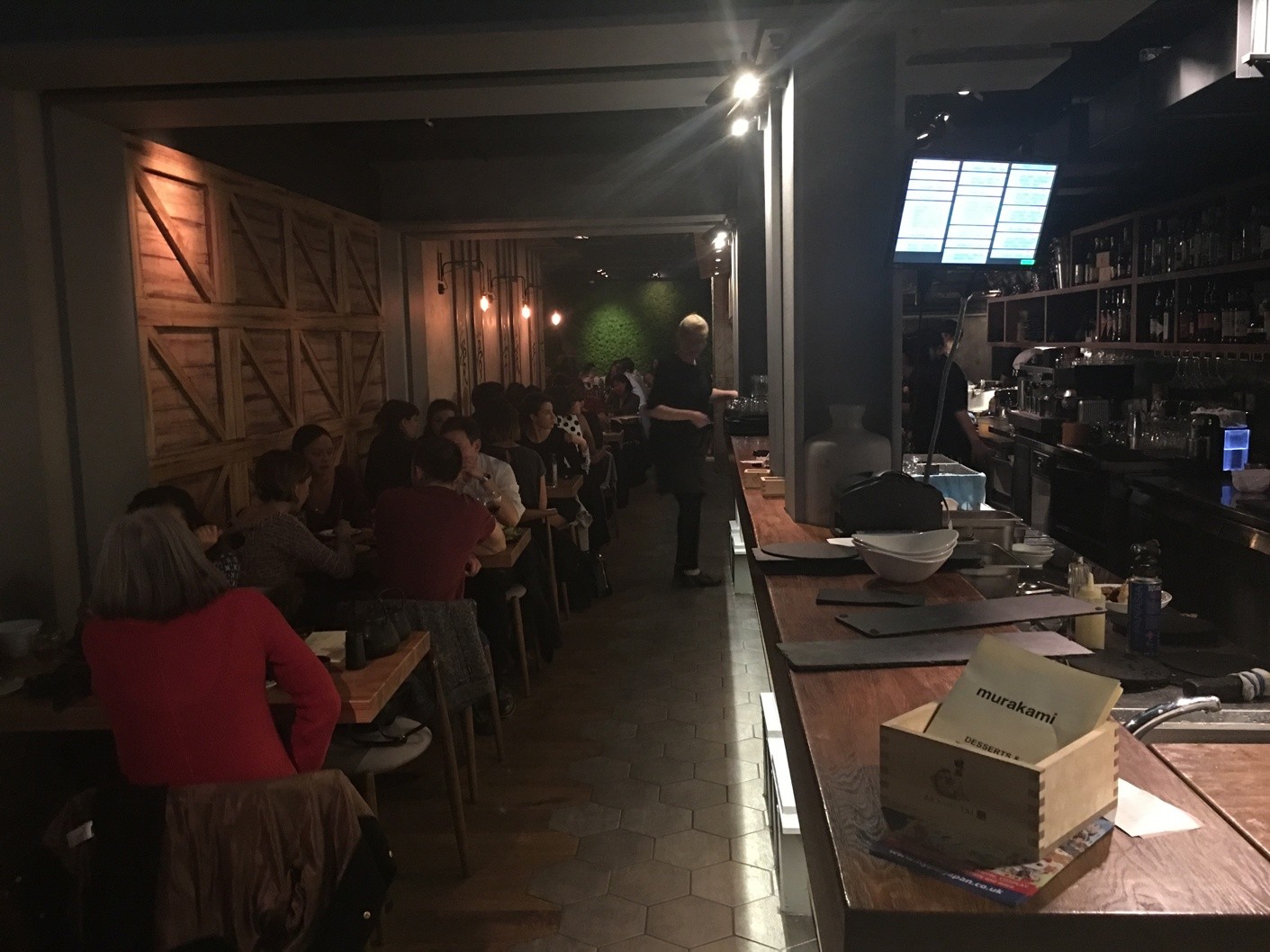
top-left (986, 172), bottom-right (1270, 457)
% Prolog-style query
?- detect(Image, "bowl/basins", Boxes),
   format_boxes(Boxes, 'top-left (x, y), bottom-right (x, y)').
top-left (1231, 469), bottom-right (1270, 492)
top-left (852, 529), bottom-right (959, 582)
top-left (1012, 543), bottom-right (1054, 568)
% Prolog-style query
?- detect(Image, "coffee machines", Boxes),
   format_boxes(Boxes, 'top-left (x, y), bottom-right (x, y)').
top-left (1006, 345), bottom-right (1136, 436)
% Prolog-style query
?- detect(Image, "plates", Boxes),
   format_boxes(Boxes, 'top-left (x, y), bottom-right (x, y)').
top-left (1094, 583), bottom-right (1173, 613)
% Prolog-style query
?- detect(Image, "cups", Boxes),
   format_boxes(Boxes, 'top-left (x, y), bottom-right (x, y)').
top-left (726, 392), bottom-right (768, 424)
top-left (483, 492), bottom-right (502, 514)
top-left (1092, 400), bottom-right (1201, 450)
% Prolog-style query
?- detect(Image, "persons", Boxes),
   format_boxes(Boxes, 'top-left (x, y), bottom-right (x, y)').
top-left (643, 313), bottom-right (739, 590)
top-left (80, 353), bottom-right (660, 951)
top-left (908, 320), bottom-right (994, 469)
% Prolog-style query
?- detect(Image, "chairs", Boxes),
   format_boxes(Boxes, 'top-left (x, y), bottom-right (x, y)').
top-left (72, 764), bottom-right (373, 952)
top-left (520, 507), bottom-right (571, 628)
top-left (330, 598), bottom-right (506, 797)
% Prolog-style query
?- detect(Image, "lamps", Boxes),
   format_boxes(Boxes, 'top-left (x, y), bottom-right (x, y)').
top-left (723, 40), bottom-right (793, 109)
top-left (439, 252), bottom-right (493, 312)
top-left (489, 268), bottom-right (534, 320)
top-left (526, 283), bottom-right (564, 326)
top-left (711, 215), bottom-right (737, 254)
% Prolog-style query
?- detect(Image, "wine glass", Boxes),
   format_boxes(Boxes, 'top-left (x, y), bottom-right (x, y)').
top-left (1172, 349), bottom-right (1227, 388)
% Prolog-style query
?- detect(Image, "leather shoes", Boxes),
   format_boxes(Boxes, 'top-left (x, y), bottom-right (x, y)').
top-left (672, 571), bottom-right (722, 587)
top-left (483, 688), bottom-right (516, 720)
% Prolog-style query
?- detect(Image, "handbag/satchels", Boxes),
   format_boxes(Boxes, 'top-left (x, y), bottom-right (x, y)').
top-left (335, 700), bottom-right (436, 745)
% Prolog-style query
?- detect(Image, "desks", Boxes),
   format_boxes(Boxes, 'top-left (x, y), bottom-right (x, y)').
top-left (319, 422), bottom-right (626, 570)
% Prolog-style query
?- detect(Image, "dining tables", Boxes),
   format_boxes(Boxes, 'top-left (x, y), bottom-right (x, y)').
top-left (1, 629), bottom-right (476, 946)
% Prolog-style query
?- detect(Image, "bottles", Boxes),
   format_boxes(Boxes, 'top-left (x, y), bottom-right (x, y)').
top-left (1075, 202), bottom-right (1269, 344)
top-left (1126, 540), bottom-right (1163, 656)
top-left (1075, 572), bottom-right (1105, 649)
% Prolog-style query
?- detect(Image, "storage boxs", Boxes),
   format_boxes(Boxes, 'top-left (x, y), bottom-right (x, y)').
top-left (902, 453), bottom-right (987, 511)
top-left (946, 540), bottom-right (1031, 598)
top-left (724, 415), bottom-right (769, 436)
top-left (942, 509), bottom-right (1024, 556)
top-left (878, 700), bottom-right (1121, 865)
